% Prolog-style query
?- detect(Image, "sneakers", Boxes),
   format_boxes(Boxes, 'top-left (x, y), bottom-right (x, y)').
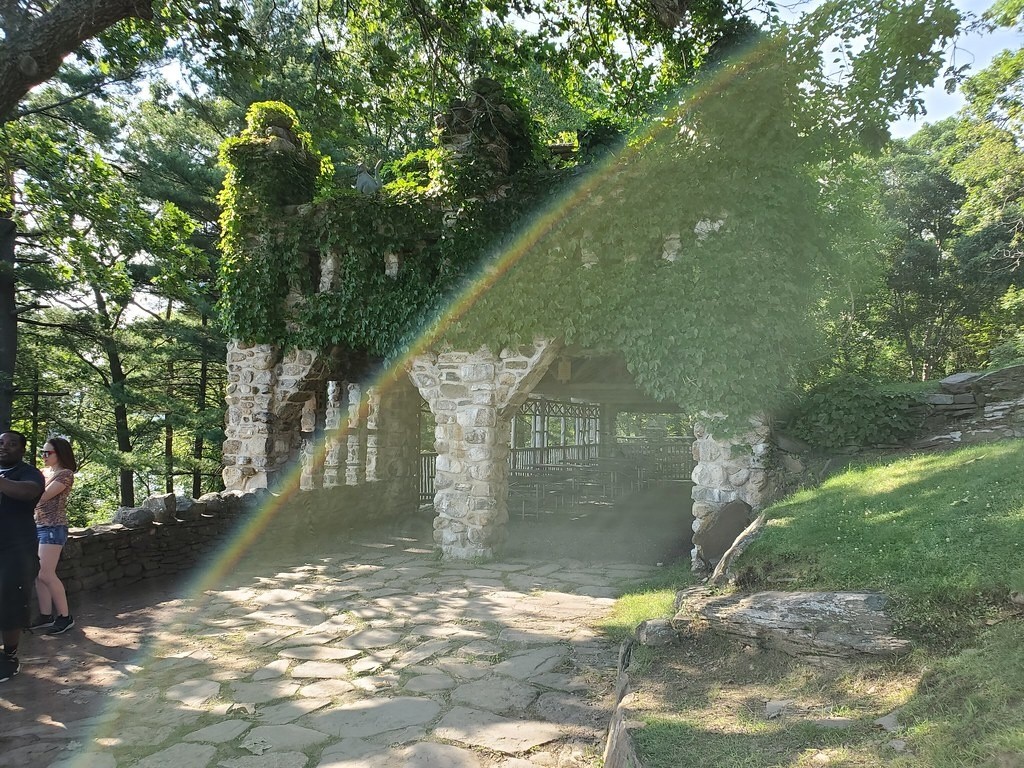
top-left (25, 614), bottom-right (54, 630)
top-left (48, 616), bottom-right (74, 635)
top-left (0, 660), bottom-right (21, 683)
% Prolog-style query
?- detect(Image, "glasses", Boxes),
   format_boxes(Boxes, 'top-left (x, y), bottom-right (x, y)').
top-left (40, 449), bottom-right (56, 457)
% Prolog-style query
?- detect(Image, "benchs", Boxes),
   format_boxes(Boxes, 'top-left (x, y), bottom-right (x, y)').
top-left (508, 460), bottom-right (641, 520)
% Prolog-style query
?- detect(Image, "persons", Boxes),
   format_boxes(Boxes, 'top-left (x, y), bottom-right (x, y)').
top-left (0, 430), bottom-right (45, 683)
top-left (27, 438), bottom-right (77, 634)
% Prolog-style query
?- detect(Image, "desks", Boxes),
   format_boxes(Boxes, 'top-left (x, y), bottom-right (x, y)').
top-left (508, 456), bottom-right (641, 515)
